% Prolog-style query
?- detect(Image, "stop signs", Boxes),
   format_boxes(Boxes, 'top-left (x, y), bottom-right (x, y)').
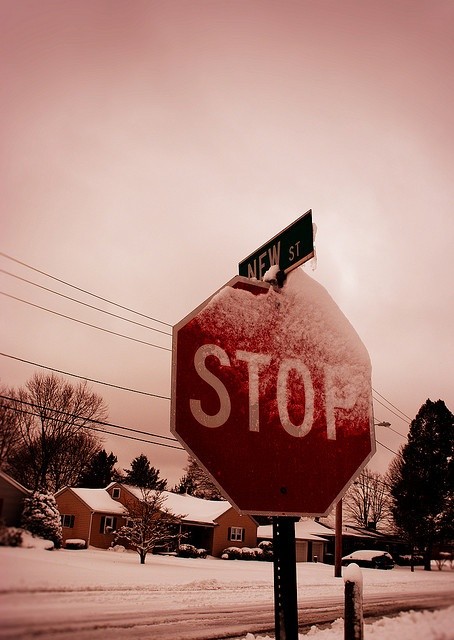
top-left (170, 275), bottom-right (376, 518)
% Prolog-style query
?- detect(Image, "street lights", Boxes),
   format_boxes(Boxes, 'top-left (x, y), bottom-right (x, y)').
top-left (334, 421), bottom-right (391, 576)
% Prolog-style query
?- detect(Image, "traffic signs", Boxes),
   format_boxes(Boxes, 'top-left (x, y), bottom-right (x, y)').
top-left (237, 209), bottom-right (314, 281)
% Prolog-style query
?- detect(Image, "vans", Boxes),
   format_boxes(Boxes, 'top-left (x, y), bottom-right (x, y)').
top-left (342, 550), bottom-right (394, 570)
